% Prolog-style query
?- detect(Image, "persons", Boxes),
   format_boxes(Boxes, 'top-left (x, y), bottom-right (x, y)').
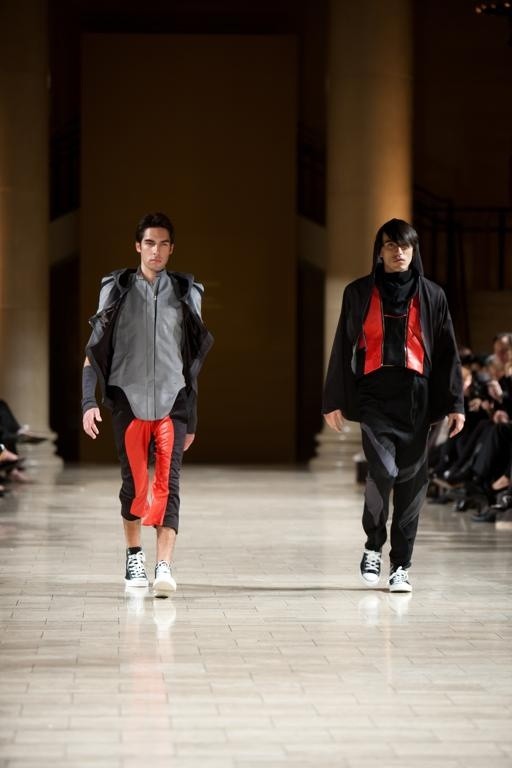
top-left (322, 219), bottom-right (466, 594)
top-left (428, 333), bottom-right (512, 523)
top-left (80, 212), bottom-right (215, 600)
top-left (0, 398), bottom-right (56, 497)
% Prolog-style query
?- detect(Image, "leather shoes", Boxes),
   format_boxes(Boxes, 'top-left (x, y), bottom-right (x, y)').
top-left (424, 464), bottom-right (512, 522)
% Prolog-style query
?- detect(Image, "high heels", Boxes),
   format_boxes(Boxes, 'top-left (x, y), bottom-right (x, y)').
top-left (1, 435), bottom-right (47, 496)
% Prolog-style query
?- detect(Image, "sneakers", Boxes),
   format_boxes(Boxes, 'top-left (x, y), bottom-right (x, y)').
top-left (389, 563), bottom-right (411, 593)
top-left (360, 549), bottom-right (382, 587)
top-left (152, 560), bottom-right (177, 598)
top-left (124, 546), bottom-right (149, 587)
